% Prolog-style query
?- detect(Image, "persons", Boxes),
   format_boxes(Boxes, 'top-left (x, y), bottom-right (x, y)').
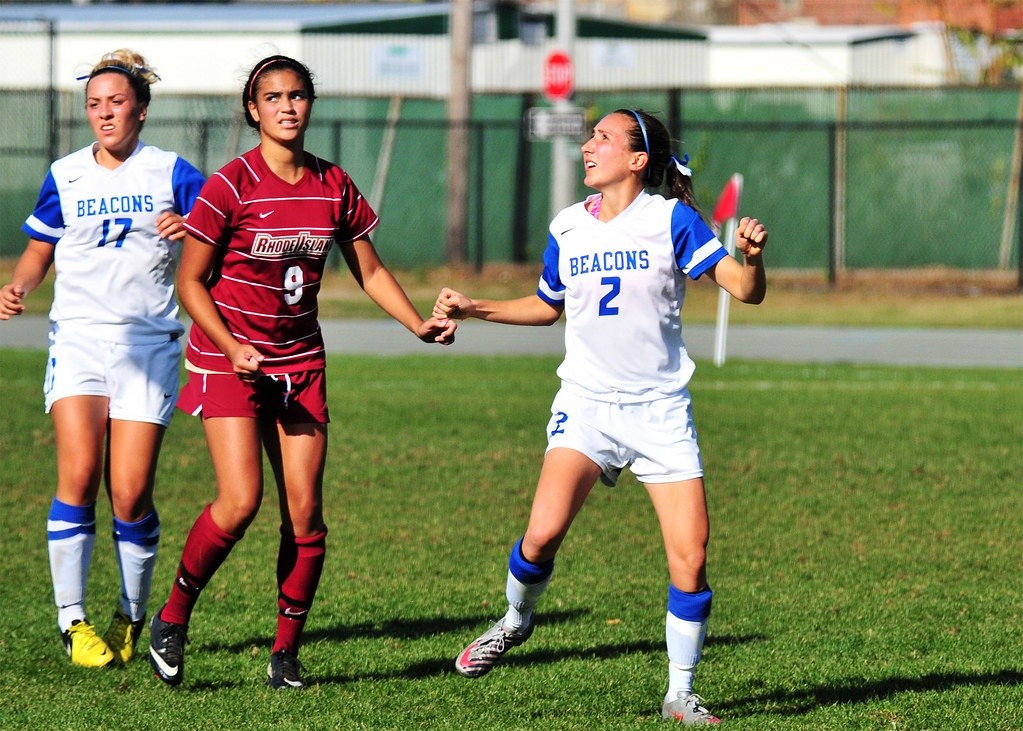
top-left (146, 55), bottom-right (458, 685)
top-left (1, 46), bottom-right (216, 675)
top-left (431, 106), bottom-right (771, 730)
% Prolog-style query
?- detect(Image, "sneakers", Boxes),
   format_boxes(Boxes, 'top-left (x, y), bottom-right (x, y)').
top-left (266, 647), bottom-right (305, 691)
top-left (58, 617), bottom-right (116, 668)
top-left (455, 615), bottom-right (535, 679)
top-left (147, 598), bottom-right (191, 686)
top-left (661, 689), bottom-right (721, 727)
top-left (103, 600), bottom-right (147, 666)
